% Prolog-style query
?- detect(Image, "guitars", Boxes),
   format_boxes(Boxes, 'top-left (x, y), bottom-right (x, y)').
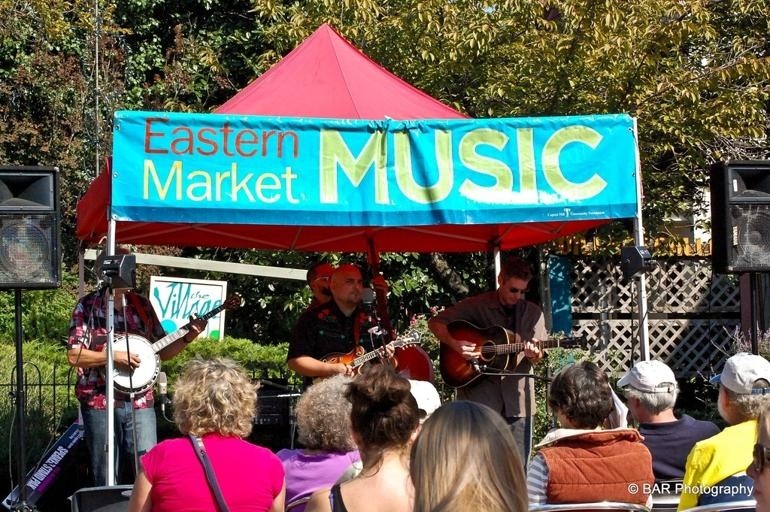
top-left (311, 329), bottom-right (421, 385)
top-left (441, 320), bottom-right (590, 387)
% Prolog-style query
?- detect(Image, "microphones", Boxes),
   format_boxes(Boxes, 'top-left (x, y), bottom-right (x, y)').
top-left (471, 358), bottom-right (482, 374)
top-left (361, 287), bottom-right (375, 324)
top-left (156, 372), bottom-right (168, 412)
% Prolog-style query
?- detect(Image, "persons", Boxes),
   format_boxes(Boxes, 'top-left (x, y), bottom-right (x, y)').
top-left (737, 390), bottom-right (770, 512)
top-left (408, 399), bottom-right (530, 512)
top-left (126, 354), bottom-right (288, 512)
top-left (274, 374), bottom-right (365, 512)
top-left (304, 364), bottom-right (423, 512)
top-left (285, 264), bottom-right (399, 393)
top-left (614, 360), bottom-right (723, 491)
top-left (427, 255), bottom-right (550, 474)
top-left (66, 246), bottom-right (209, 487)
top-left (302, 261), bottom-right (393, 330)
top-left (524, 359), bottom-right (656, 511)
top-left (672, 350), bottom-right (770, 512)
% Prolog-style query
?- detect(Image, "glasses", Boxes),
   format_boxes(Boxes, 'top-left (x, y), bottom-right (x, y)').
top-left (753, 443), bottom-right (769, 472)
top-left (509, 287), bottom-right (530, 295)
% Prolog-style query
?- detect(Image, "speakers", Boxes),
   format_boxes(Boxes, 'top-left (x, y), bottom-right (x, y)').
top-left (710, 159), bottom-right (770, 274)
top-left (0, 165), bottom-right (62, 291)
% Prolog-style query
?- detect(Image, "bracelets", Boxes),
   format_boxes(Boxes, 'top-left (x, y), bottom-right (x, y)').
top-left (373, 297), bottom-right (388, 308)
top-left (344, 363), bottom-right (349, 375)
top-left (181, 334), bottom-right (194, 344)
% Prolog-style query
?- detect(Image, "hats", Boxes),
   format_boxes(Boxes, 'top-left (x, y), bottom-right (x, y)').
top-left (709, 352), bottom-right (769, 395)
top-left (408, 379), bottom-right (441, 424)
top-left (616, 359), bottom-right (676, 393)
